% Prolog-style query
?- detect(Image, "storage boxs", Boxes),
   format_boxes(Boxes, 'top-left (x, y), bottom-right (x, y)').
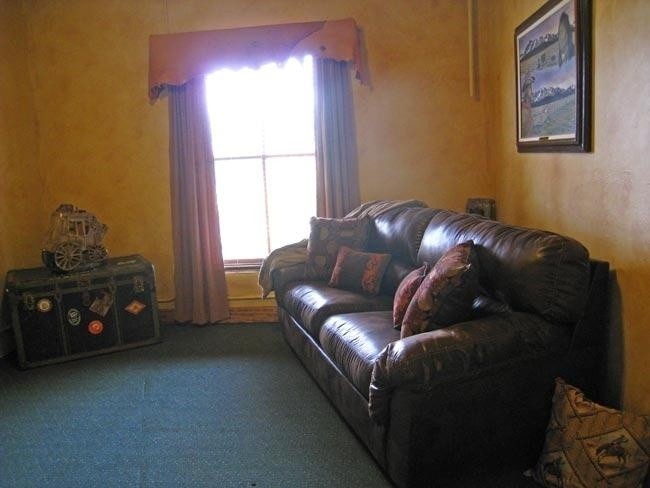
top-left (5, 254), bottom-right (163, 372)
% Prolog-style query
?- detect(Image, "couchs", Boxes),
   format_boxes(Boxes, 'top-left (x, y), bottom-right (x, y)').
top-left (271, 200), bottom-right (610, 488)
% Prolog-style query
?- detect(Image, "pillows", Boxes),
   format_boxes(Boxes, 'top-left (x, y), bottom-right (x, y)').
top-left (307, 214), bottom-right (479, 339)
top-left (524, 378), bottom-right (650, 488)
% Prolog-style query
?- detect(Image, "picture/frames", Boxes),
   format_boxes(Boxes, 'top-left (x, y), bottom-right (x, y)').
top-left (513, 0), bottom-right (593, 153)
